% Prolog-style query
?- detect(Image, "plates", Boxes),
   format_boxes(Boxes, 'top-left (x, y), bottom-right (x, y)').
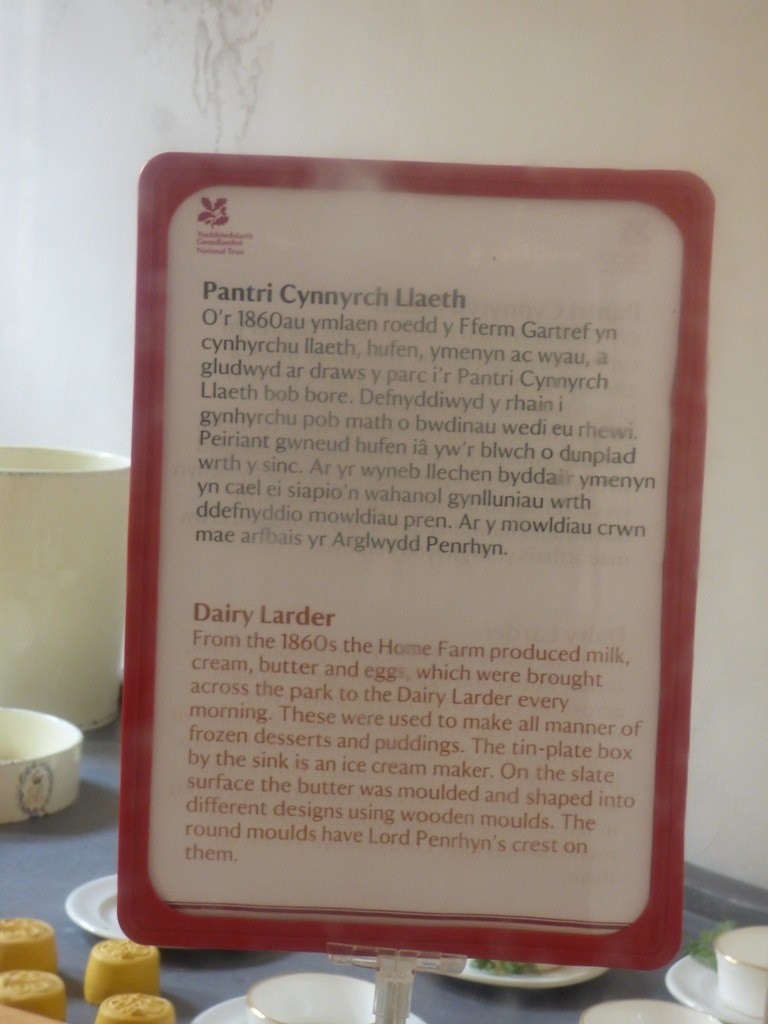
top-left (459, 958), bottom-right (610, 989)
top-left (663, 954), bottom-right (768, 1024)
top-left (64, 872), bottom-right (197, 950)
top-left (578, 998), bottom-right (721, 1024)
top-left (189, 996), bottom-right (428, 1024)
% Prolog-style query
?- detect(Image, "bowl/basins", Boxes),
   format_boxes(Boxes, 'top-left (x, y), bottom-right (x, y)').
top-left (0, 708), bottom-right (83, 825)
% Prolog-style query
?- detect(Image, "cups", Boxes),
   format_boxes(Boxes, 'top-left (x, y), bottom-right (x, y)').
top-left (713, 925), bottom-right (768, 1018)
top-left (244, 972), bottom-right (376, 1024)
